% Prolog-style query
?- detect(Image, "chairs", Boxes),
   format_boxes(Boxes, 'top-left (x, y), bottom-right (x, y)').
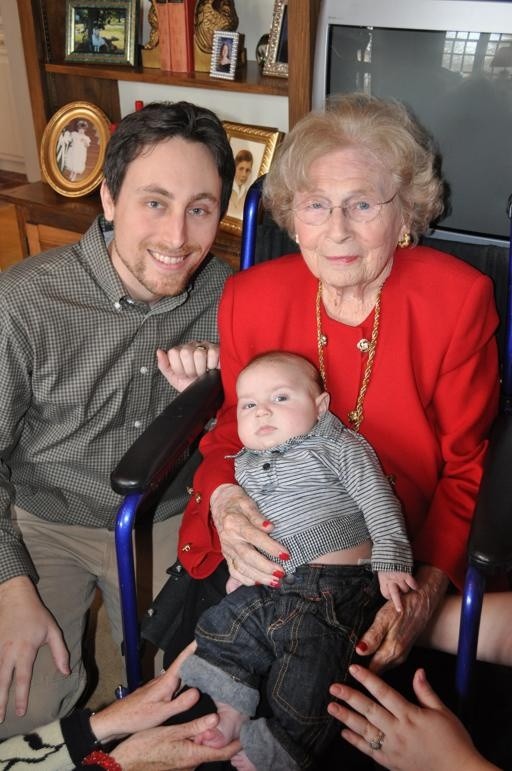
top-left (107, 175), bottom-right (510, 721)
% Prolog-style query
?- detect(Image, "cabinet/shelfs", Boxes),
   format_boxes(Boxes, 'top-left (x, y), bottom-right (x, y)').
top-left (1, 1), bottom-right (316, 277)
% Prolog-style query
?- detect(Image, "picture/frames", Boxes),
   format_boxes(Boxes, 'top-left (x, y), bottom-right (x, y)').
top-left (40, 102), bottom-right (109, 197)
top-left (264, 1), bottom-right (287, 79)
top-left (210, 30), bottom-right (239, 80)
top-left (65, 2), bottom-right (139, 69)
top-left (215, 120), bottom-right (284, 238)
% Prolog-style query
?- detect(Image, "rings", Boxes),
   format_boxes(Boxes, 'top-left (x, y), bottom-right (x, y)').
top-left (194, 346), bottom-right (207, 351)
top-left (370, 734), bottom-right (382, 750)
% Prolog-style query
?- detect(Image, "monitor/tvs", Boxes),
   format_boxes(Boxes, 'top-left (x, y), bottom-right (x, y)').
top-left (313, 0), bottom-right (511, 248)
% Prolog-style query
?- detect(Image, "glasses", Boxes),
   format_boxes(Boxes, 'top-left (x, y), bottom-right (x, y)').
top-left (290, 194), bottom-right (395, 224)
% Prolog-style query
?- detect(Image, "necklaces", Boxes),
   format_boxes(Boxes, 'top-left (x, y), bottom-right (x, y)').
top-left (311, 272), bottom-right (388, 436)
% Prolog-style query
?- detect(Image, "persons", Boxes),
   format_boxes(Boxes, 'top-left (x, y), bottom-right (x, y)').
top-left (216, 44), bottom-right (230, 73)
top-left (326, 591), bottom-right (509, 771)
top-left (176, 90), bottom-right (494, 770)
top-left (91, 26), bottom-right (106, 54)
top-left (65, 118), bottom-right (91, 183)
top-left (4, 100), bottom-right (250, 735)
top-left (2, 635), bottom-right (250, 769)
top-left (182, 353), bottom-right (415, 770)
top-left (225, 151), bottom-right (255, 220)
